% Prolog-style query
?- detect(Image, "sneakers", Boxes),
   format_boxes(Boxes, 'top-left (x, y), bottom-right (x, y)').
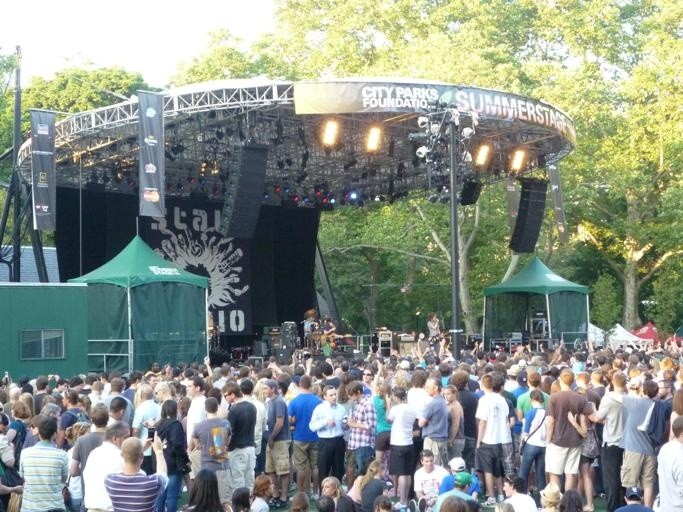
top-left (481, 498), bottom-right (503, 508)
top-left (409, 498), bottom-right (426, 512)
top-left (267, 498), bottom-right (289, 509)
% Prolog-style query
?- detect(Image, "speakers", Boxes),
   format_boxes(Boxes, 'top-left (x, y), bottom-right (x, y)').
top-left (460, 181), bottom-right (481, 206)
top-left (217, 144), bottom-right (269, 240)
top-left (509, 181), bottom-right (548, 254)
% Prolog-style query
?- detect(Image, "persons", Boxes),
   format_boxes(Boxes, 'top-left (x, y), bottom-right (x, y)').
top-left (1, 308), bottom-right (681, 511)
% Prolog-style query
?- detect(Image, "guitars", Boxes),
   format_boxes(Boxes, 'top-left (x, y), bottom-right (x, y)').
top-left (428, 331), bottom-right (451, 346)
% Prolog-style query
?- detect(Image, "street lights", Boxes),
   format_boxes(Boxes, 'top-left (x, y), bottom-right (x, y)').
top-left (413, 104), bottom-right (481, 358)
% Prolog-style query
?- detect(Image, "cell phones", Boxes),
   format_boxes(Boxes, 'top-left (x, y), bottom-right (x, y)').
top-left (147, 425), bottom-right (159, 444)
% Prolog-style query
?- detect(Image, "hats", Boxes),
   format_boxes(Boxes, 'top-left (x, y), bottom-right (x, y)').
top-left (626, 487), bottom-right (642, 499)
top-left (264, 380), bottom-right (279, 395)
top-left (507, 365), bottom-right (527, 383)
top-left (540, 483), bottom-right (563, 504)
top-left (448, 457), bottom-right (471, 487)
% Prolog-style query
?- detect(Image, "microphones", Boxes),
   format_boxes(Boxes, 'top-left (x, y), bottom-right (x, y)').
top-left (416, 310), bottom-right (420, 316)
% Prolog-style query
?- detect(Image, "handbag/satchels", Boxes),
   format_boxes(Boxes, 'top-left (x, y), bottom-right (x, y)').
top-left (520, 438), bottom-right (527, 455)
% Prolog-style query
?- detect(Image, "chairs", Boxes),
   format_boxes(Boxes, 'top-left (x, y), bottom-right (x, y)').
top-left (338, 335), bottom-right (359, 351)
